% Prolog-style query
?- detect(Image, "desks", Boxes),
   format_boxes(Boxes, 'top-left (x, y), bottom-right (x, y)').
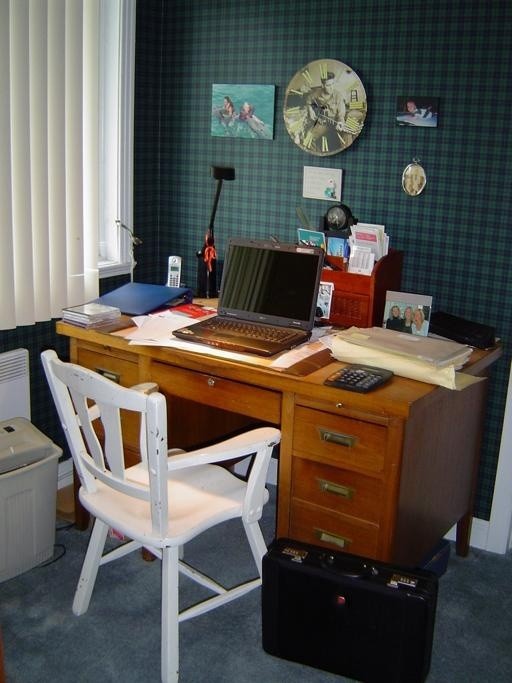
top-left (54, 297), bottom-right (502, 569)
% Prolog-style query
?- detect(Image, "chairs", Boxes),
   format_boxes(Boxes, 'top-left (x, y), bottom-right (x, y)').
top-left (40, 350), bottom-right (283, 681)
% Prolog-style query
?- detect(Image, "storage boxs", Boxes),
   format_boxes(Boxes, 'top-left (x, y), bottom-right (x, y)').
top-left (318, 246), bottom-right (402, 328)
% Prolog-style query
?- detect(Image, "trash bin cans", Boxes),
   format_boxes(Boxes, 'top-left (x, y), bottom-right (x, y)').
top-left (0, 417), bottom-right (63, 583)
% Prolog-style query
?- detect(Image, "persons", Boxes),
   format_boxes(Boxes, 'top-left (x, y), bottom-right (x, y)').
top-left (400, 305), bottom-right (414, 333)
top-left (232, 101), bottom-right (267, 137)
top-left (411, 308), bottom-right (429, 336)
top-left (405, 99), bottom-right (436, 119)
top-left (386, 305), bottom-right (404, 331)
top-left (304, 70), bottom-right (346, 151)
top-left (218, 94), bottom-right (237, 126)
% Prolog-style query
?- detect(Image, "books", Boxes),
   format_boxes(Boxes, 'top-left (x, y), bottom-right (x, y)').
top-left (331, 324), bottom-right (474, 374)
top-left (61, 301), bottom-right (121, 328)
top-left (297, 221), bottom-right (390, 262)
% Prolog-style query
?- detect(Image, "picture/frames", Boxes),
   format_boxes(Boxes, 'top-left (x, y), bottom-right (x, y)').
top-left (382, 290), bottom-right (433, 337)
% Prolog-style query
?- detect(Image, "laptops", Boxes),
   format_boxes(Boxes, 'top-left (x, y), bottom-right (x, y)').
top-left (172, 237), bottom-right (326, 357)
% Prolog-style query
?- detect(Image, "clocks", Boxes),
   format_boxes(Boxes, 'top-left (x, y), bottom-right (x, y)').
top-left (319, 203), bottom-right (356, 237)
top-left (283, 58), bottom-right (368, 157)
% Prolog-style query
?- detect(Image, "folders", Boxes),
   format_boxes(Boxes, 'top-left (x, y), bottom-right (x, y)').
top-left (89, 283), bottom-right (193, 315)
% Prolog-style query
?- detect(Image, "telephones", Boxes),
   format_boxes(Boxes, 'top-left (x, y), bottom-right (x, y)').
top-left (165, 255), bottom-right (186, 288)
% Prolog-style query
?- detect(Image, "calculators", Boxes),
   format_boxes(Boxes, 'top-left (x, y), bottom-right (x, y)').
top-left (324, 363), bottom-right (394, 394)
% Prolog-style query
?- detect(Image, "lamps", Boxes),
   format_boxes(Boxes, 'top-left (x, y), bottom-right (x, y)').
top-left (197, 165), bottom-right (236, 297)
